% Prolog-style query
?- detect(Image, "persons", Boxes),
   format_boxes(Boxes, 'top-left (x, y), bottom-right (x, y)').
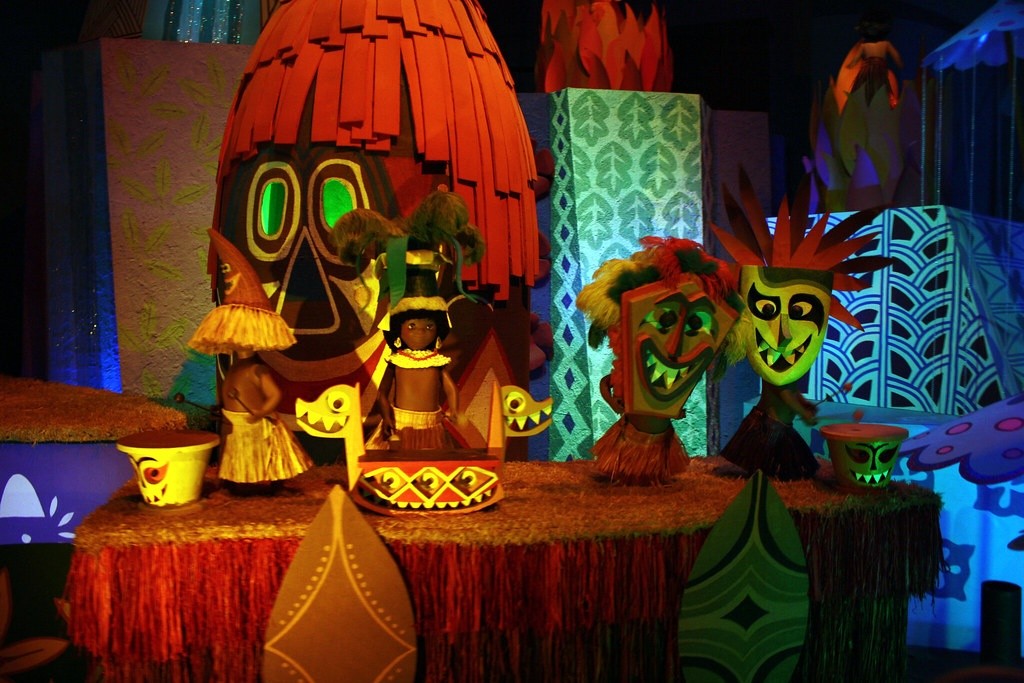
top-left (369, 252), bottom-right (466, 453)
top-left (188, 227), bottom-right (313, 493)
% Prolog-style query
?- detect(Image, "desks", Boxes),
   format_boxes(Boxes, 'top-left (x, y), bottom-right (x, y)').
top-left (65, 460), bottom-right (948, 683)
